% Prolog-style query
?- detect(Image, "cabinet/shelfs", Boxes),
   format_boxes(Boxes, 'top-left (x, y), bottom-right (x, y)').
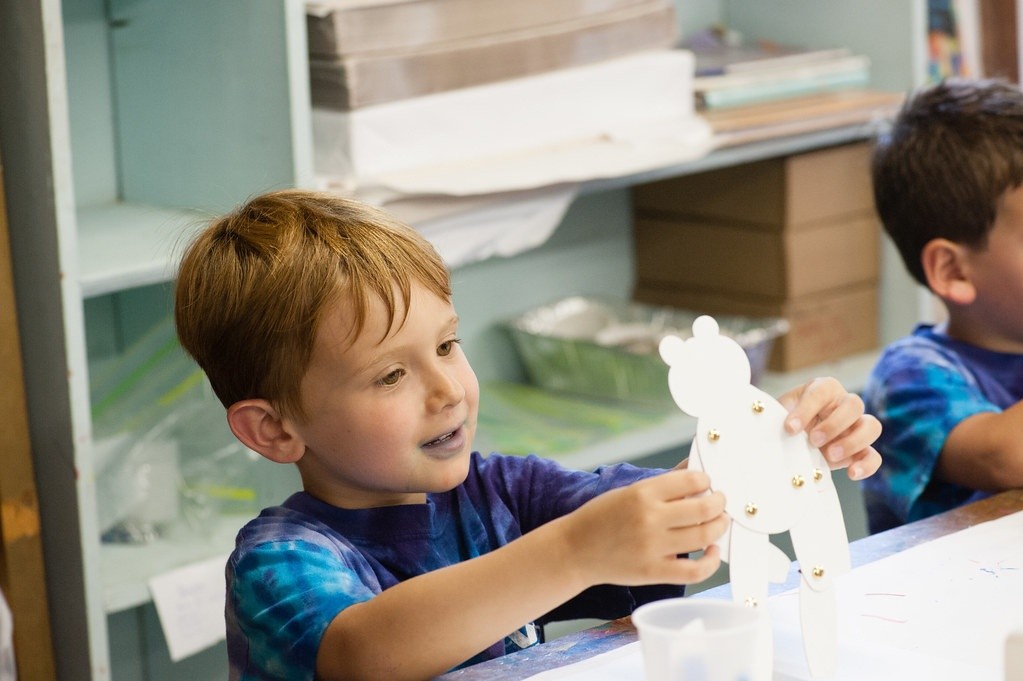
top-left (0, 0), bottom-right (932, 680)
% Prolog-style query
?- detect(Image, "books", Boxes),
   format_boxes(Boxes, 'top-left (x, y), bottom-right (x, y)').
top-left (687, 42), bottom-right (904, 146)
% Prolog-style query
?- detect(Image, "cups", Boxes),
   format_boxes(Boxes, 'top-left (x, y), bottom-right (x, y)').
top-left (631, 596), bottom-right (781, 681)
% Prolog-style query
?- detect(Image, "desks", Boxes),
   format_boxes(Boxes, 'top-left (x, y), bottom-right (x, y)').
top-left (441, 489), bottom-right (1023, 680)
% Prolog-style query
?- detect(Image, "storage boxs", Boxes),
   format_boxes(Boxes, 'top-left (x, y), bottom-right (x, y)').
top-left (497, 135), bottom-right (879, 412)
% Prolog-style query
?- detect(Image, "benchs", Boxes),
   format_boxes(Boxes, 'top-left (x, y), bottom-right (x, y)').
top-left (101, 386), bottom-right (865, 679)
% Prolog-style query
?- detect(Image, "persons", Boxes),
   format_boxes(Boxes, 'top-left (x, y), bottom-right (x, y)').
top-left (862, 78), bottom-right (1023, 535)
top-left (174, 191), bottom-right (882, 680)
